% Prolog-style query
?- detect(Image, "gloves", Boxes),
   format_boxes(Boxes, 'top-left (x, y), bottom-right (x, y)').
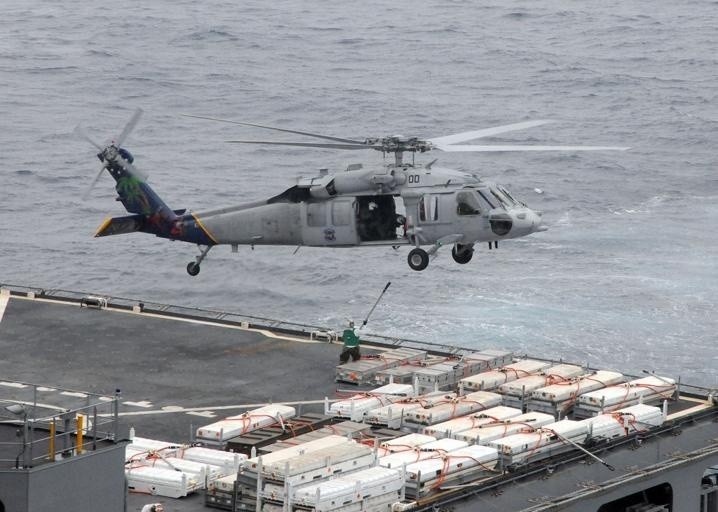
top-left (368, 202), bottom-right (378, 211)
top-left (397, 215), bottom-right (406, 224)
top-left (363, 320), bottom-right (367, 325)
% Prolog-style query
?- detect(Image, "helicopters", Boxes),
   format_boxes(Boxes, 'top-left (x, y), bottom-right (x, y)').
top-left (81, 105), bottom-right (631, 276)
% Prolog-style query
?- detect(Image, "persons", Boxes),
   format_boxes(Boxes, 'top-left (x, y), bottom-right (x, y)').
top-left (339, 320), bottom-right (367, 368)
